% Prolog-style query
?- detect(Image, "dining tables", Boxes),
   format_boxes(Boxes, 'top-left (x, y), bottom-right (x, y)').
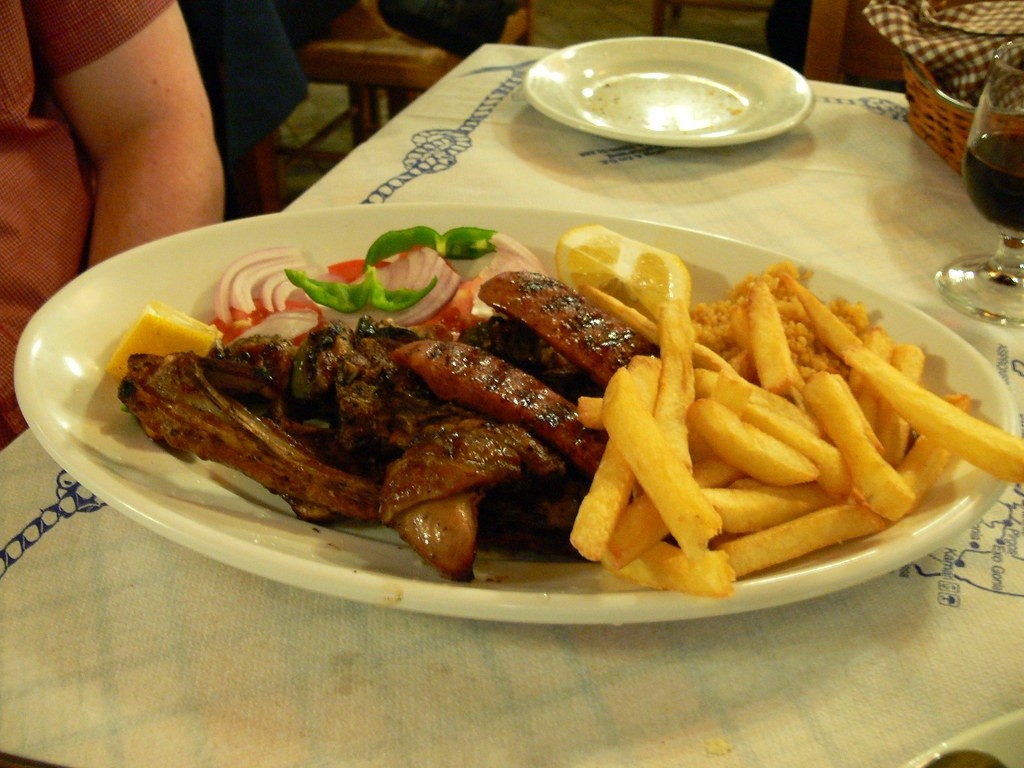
top-left (1, 43), bottom-right (1022, 768)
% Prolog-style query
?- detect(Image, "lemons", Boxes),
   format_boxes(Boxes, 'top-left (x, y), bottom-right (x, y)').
top-left (556, 221), bottom-right (690, 318)
top-left (107, 306), bottom-right (221, 383)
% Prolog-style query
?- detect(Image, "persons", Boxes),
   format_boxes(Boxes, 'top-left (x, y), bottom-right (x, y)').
top-left (0, 0), bottom-right (227, 448)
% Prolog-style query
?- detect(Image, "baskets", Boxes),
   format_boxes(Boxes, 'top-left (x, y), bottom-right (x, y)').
top-left (899, 52), bottom-right (1023, 176)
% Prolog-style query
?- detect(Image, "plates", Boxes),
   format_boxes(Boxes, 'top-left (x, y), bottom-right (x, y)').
top-left (524, 35), bottom-right (813, 148)
top-left (11, 197), bottom-right (1019, 621)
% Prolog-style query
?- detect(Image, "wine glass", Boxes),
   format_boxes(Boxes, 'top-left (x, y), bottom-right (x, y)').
top-left (935, 38), bottom-right (1024, 329)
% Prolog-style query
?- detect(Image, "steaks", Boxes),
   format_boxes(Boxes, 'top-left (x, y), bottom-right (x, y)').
top-left (122, 277), bottom-right (658, 591)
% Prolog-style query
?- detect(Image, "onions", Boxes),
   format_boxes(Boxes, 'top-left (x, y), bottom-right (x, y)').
top-left (214, 232), bottom-right (539, 343)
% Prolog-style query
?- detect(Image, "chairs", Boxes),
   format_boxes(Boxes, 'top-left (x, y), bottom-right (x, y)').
top-left (240, 0), bottom-right (537, 214)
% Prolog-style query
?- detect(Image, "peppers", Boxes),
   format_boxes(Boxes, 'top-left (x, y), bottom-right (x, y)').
top-left (364, 225), bottom-right (499, 263)
top-left (283, 268), bottom-right (439, 314)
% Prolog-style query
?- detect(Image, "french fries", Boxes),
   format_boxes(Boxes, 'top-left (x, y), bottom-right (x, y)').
top-left (574, 276), bottom-right (1024, 596)
